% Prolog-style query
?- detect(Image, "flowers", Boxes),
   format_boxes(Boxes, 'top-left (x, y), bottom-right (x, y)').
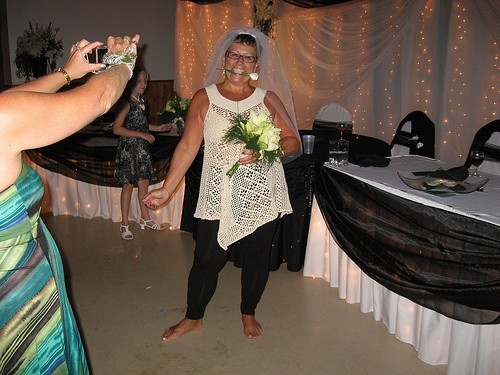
top-left (221, 107), bottom-right (285, 178)
top-left (155, 94), bottom-right (191, 134)
top-left (13, 18), bottom-right (67, 82)
top-left (252, 0), bottom-right (279, 39)
top-left (216, 68), bottom-right (259, 82)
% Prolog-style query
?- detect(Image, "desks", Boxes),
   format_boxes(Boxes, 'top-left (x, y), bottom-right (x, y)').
top-left (21, 120), bottom-right (500, 375)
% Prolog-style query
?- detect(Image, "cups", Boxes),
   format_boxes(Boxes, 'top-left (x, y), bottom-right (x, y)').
top-left (303, 135), bottom-right (315, 154)
top-left (329, 139), bottom-right (349, 167)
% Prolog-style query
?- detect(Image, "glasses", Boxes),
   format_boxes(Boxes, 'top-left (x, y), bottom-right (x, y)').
top-left (225, 51), bottom-right (257, 63)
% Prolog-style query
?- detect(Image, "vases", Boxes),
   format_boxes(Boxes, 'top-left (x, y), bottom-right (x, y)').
top-left (30, 58), bottom-right (57, 79)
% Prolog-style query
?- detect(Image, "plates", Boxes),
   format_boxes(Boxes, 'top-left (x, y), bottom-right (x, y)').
top-left (397, 167), bottom-right (490, 193)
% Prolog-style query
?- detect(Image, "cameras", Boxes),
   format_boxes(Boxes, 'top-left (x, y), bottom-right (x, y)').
top-left (96, 46), bottom-right (109, 67)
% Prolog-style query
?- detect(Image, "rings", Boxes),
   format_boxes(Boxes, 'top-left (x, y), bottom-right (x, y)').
top-left (131, 41), bottom-right (138, 46)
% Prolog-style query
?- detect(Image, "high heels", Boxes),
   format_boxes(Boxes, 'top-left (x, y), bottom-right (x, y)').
top-left (139, 218), bottom-right (165, 230)
top-left (119, 224), bottom-right (133, 240)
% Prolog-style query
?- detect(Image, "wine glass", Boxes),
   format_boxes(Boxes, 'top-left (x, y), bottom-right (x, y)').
top-left (470, 151), bottom-right (485, 178)
top-left (337, 121), bottom-right (347, 141)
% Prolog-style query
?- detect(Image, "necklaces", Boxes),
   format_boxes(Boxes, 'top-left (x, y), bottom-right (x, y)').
top-left (130, 94), bottom-right (144, 103)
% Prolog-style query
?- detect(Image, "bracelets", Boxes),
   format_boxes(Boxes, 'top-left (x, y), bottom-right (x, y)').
top-left (57, 67), bottom-right (71, 86)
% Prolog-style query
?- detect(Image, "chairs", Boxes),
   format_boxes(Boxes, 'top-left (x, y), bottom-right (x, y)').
top-left (389, 111), bottom-right (435, 159)
top-left (311, 102), bottom-right (353, 133)
top-left (462, 119), bottom-right (500, 180)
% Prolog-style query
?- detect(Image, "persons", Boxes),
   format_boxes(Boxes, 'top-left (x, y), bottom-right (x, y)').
top-left (0, 34), bottom-right (140, 375)
top-left (113, 66), bottom-right (173, 240)
top-left (141, 27), bottom-right (302, 341)
